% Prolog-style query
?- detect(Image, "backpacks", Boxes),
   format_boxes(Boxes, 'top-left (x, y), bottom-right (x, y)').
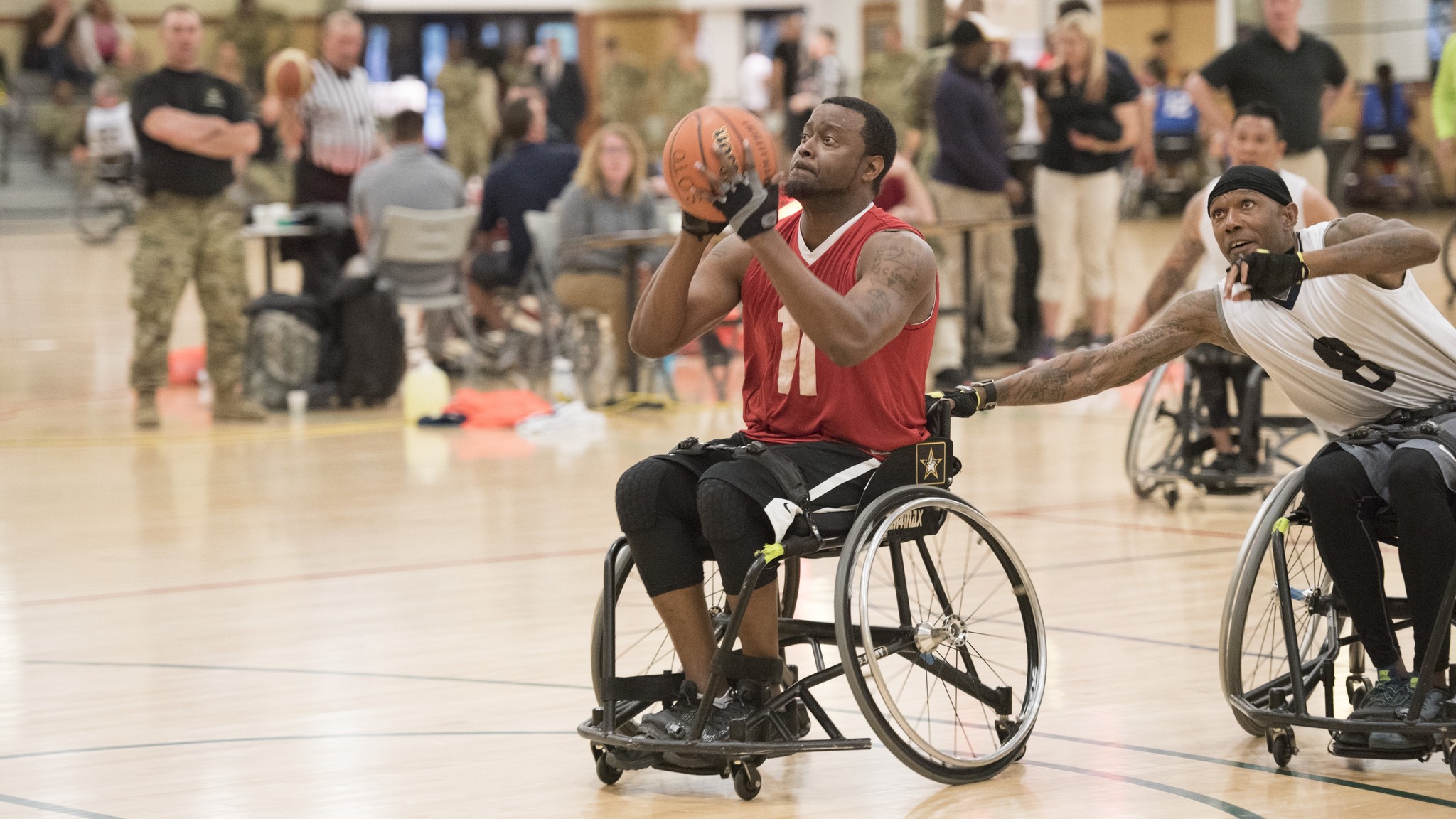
top-left (331, 277), bottom-right (406, 396)
top-left (249, 311), bottom-right (320, 409)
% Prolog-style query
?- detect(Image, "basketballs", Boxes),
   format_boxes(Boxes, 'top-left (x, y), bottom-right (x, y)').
top-left (662, 104), bottom-right (777, 223)
top-left (264, 45), bottom-right (319, 101)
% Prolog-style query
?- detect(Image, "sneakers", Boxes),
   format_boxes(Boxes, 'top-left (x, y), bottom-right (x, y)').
top-left (663, 681), bottom-right (800, 769)
top-left (1338, 668), bottom-right (1410, 745)
top-left (605, 681), bottom-right (722, 772)
top-left (1370, 671), bottom-right (1450, 751)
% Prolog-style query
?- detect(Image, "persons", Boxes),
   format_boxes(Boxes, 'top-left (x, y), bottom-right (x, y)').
top-left (604, 95), bottom-right (940, 771)
top-left (927, 165), bottom-right (1456, 720)
top-left (0, 0), bottom-right (1456, 482)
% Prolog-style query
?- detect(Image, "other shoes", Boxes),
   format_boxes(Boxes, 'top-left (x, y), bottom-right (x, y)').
top-left (137, 394), bottom-right (159, 427)
top-left (211, 396), bottom-right (265, 422)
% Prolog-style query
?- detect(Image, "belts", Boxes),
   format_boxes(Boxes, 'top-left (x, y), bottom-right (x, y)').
top-left (1284, 137), bottom-right (1321, 154)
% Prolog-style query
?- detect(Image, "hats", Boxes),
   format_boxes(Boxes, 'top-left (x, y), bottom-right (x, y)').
top-left (952, 11), bottom-right (1003, 48)
top-left (1207, 166), bottom-right (1294, 217)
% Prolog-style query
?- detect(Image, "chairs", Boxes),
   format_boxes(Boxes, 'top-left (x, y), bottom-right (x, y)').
top-left (355, 205), bottom-right (578, 396)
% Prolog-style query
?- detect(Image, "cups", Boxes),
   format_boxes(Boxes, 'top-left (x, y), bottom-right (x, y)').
top-left (287, 390), bottom-right (307, 422)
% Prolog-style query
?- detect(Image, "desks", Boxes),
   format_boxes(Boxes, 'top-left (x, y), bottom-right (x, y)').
top-left (581, 212), bottom-right (1047, 389)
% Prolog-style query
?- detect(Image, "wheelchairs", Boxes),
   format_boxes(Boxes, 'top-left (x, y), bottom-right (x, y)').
top-left (1332, 135), bottom-right (1451, 220)
top-left (70, 160), bottom-right (151, 246)
top-left (1117, 140), bottom-right (1225, 220)
top-left (577, 397), bottom-right (1049, 803)
top-left (361, 204), bottom-right (719, 408)
top-left (1217, 460), bottom-right (1456, 778)
top-left (1123, 343), bottom-right (1322, 502)
